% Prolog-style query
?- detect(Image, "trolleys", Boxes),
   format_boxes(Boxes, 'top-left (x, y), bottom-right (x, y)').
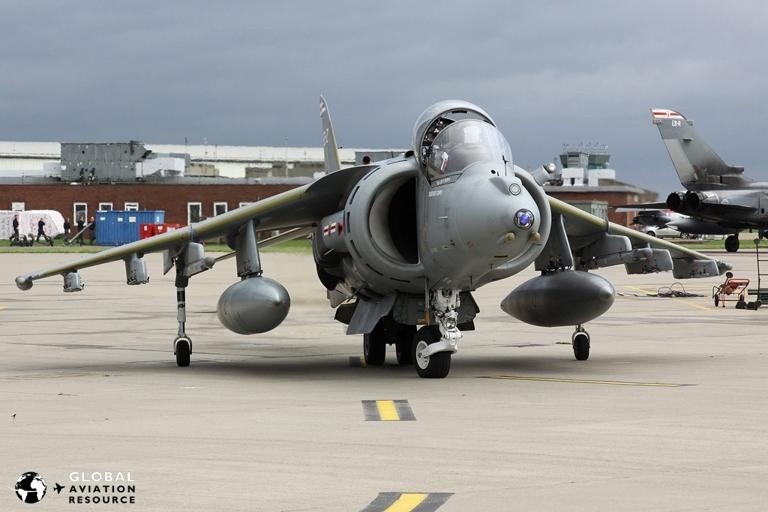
top-left (709, 278), bottom-right (752, 308)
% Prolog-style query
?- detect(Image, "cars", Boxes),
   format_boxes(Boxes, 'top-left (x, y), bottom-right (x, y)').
top-left (640, 224), bottom-right (690, 239)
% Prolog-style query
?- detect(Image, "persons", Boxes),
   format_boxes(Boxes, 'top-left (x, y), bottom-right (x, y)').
top-left (9, 214), bottom-right (96, 245)
top-left (79, 167), bottom-right (96, 183)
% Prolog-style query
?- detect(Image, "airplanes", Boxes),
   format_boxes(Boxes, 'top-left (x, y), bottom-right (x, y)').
top-left (10, 94), bottom-right (737, 379)
top-left (608, 99), bottom-right (767, 253)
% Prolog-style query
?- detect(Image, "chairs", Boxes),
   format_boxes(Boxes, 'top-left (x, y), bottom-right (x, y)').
top-left (712, 278), bottom-right (750, 306)
